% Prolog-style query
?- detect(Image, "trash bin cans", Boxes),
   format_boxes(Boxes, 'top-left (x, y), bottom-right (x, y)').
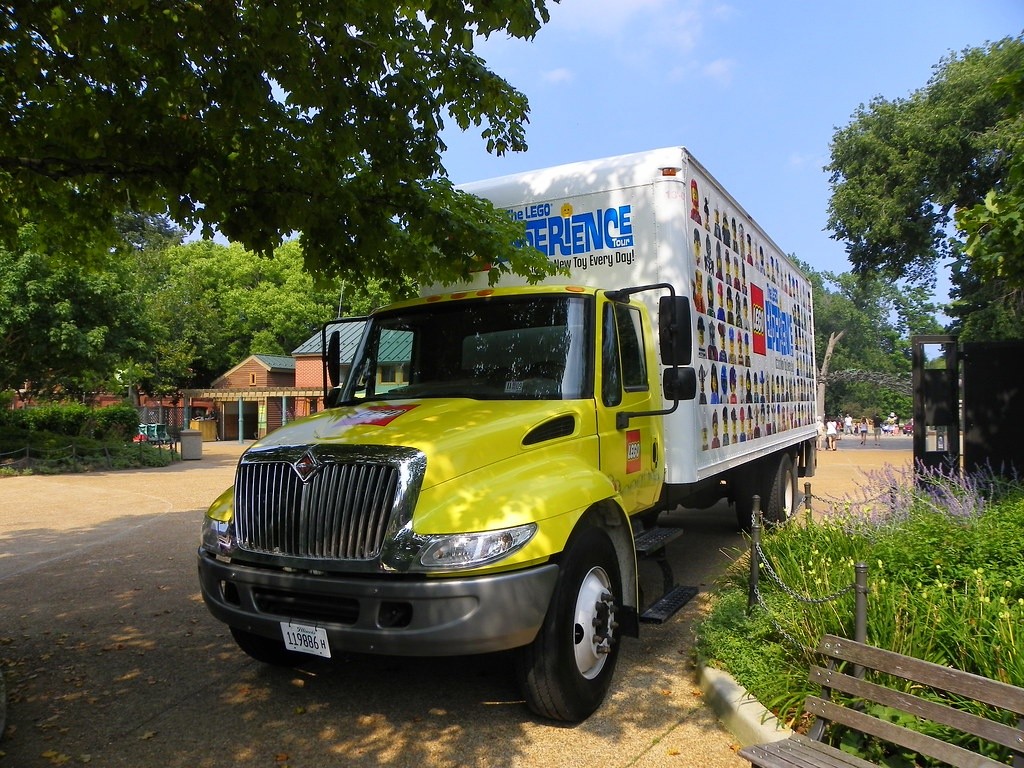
top-left (180, 429), bottom-right (203, 459)
top-left (138, 423), bottom-right (166, 444)
top-left (189, 420), bottom-right (217, 441)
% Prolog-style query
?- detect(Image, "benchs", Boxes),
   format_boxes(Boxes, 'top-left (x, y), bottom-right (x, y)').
top-left (736, 633), bottom-right (1024, 768)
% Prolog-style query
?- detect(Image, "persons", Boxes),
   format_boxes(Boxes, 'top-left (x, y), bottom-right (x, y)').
top-left (872, 413), bottom-right (882, 445)
top-left (887, 412), bottom-right (898, 436)
top-left (858, 417), bottom-right (868, 445)
top-left (826, 414), bottom-right (853, 450)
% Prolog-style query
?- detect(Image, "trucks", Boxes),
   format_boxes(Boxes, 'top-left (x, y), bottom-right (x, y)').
top-left (192, 143), bottom-right (822, 726)
